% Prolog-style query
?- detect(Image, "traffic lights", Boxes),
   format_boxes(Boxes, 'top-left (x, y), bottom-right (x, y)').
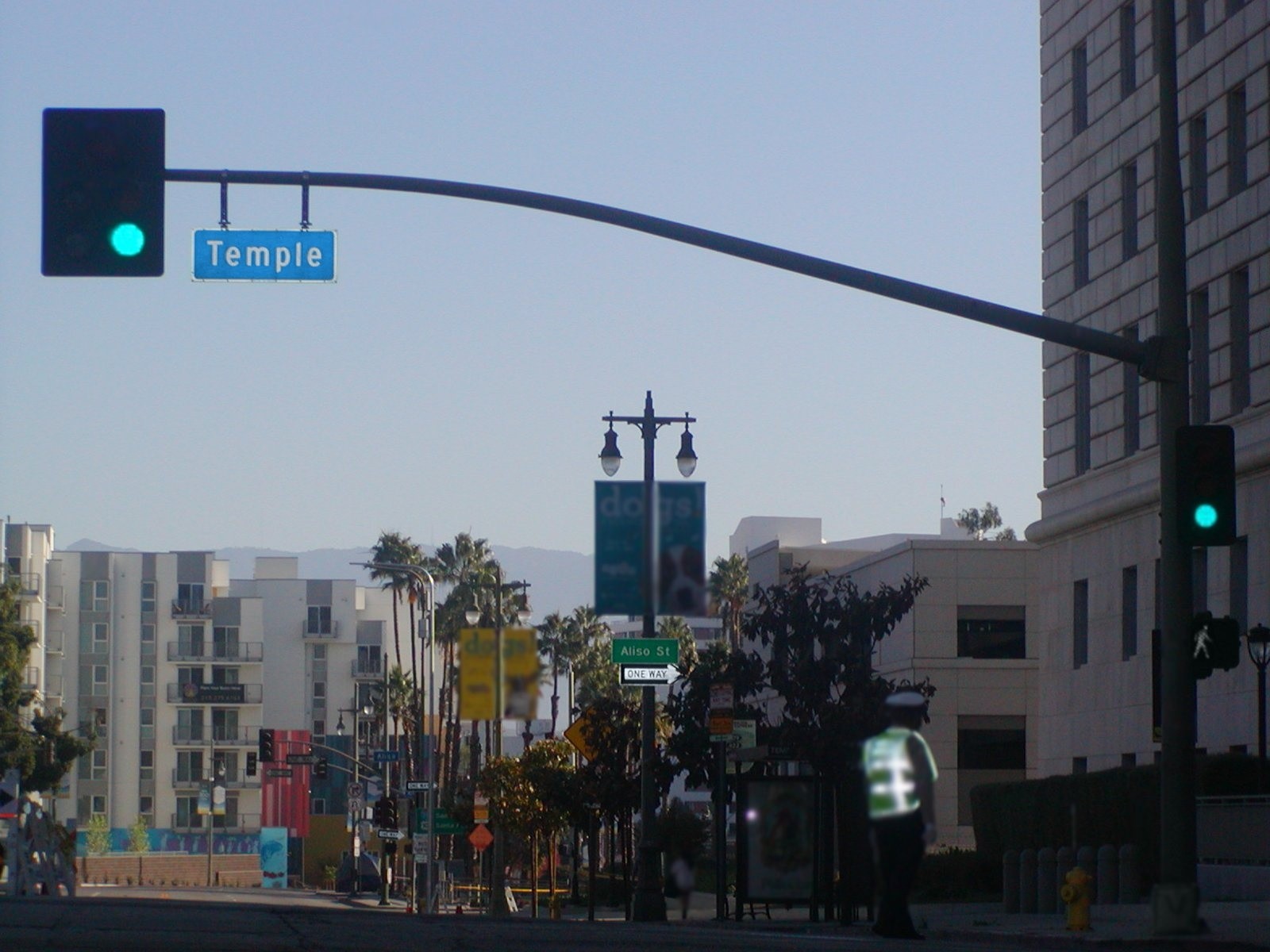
top-left (383, 797), bottom-right (397, 829)
top-left (316, 759), bottom-right (328, 780)
top-left (1189, 614), bottom-right (1218, 671)
top-left (41, 108), bottom-right (164, 275)
top-left (259, 729), bottom-right (275, 761)
top-left (1179, 424), bottom-right (1236, 545)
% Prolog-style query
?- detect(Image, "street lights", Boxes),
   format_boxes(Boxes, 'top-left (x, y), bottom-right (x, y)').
top-left (597, 390), bottom-right (697, 922)
top-left (336, 681), bottom-right (377, 898)
top-left (465, 565), bottom-right (531, 916)
top-left (1246, 621), bottom-right (1270, 792)
top-left (207, 739), bottom-right (227, 887)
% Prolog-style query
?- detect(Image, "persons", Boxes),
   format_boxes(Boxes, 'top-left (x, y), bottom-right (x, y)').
top-left (858, 691), bottom-right (936, 940)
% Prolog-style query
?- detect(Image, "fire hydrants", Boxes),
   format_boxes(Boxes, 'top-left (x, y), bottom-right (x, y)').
top-left (1060, 866), bottom-right (1097, 930)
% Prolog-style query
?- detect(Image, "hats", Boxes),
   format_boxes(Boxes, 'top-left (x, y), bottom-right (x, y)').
top-left (884, 693), bottom-right (926, 710)
